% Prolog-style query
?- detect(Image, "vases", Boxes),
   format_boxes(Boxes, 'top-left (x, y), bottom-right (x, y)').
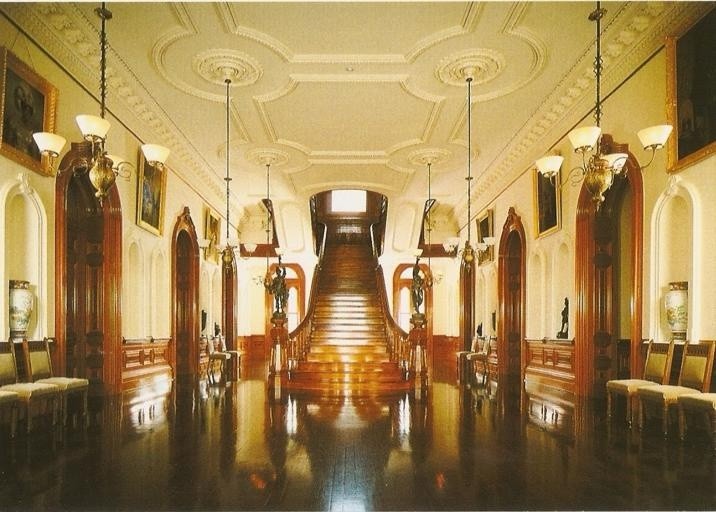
top-left (663, 282), bottom-right (689, 340)
top-left (9, 280), bottom-right (34, 343)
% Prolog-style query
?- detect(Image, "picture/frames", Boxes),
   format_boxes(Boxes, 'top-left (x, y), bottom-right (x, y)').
top-left (0, 46), bottom-right (61, 178)
top-left (136, 152), bottom-right (168, 239)
top-left (475, 209), bottom-right (496, 268)
top-left (204, 207), bottom-right (222, 266)
top-left (663, 2), bottom-right (716, 175)
top-left (532, 148), bottom-right (563, 241)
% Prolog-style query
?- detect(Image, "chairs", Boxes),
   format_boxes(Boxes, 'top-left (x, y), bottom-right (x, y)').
top-left (455, 336), bottom-right (492, 375)
top-left (206, 335), bottom-right (239, 371)
top-left (605, 338), bottom-right (716, 451)
top-left (0, 337), bottom-right (90, 441)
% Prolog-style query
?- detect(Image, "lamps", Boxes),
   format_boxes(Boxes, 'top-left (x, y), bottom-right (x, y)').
top-left (534, 1), bottom-right (674, 212)
top-left (32, 1), bottom-right (171, 208)
top-left (196, 66), bottom-right (258, 274)
top-left (441, 68), bottom-right (497, 276)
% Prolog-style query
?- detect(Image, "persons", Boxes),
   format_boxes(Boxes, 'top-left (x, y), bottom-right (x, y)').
top-left (266, 264), bottom-right (289, 314)
top-left (9, 84), bottom-right (39, 157)
top-left (409, 255), bottom-right (427, 315)
